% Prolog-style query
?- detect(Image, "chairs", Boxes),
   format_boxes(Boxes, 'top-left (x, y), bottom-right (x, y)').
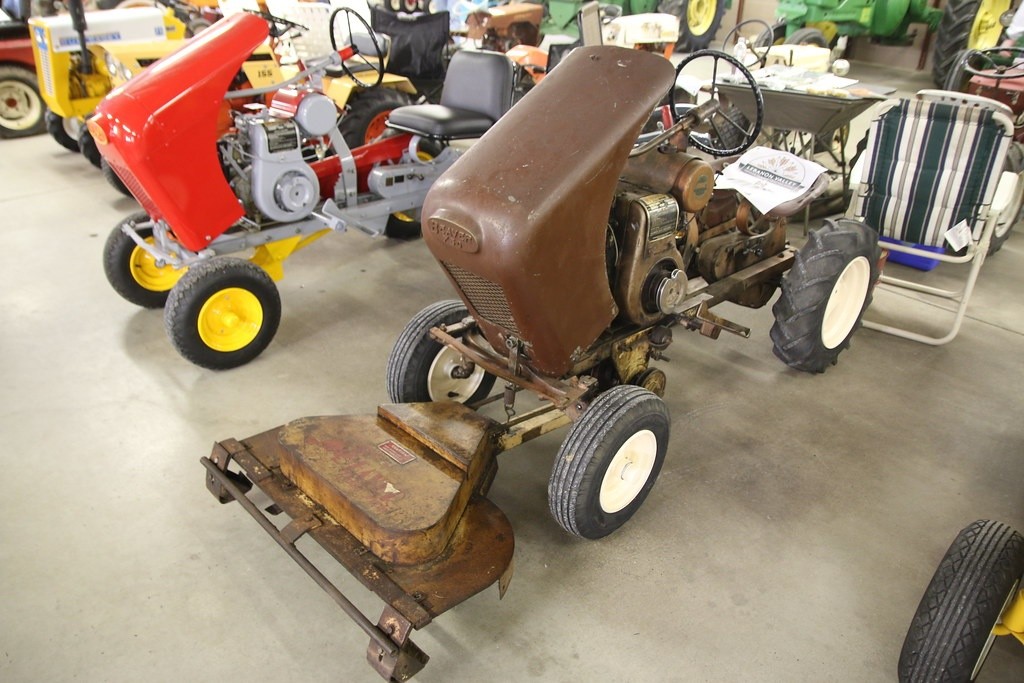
top-left (846, 99), bottom-right (1013, 346)
top-left (383, 49), bottom-right (519, 165)
top-left (305, 33), bottom-right (392, 110)
top-left (578, 0), bottom-right (699, 134)
top-left (917, 88), bottom-right (1024, 257)
top-left (366, 0), bottom-right (449, 100)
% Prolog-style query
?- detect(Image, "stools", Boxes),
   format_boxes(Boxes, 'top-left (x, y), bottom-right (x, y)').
top-left (708, 154), bottom-right (829, 237)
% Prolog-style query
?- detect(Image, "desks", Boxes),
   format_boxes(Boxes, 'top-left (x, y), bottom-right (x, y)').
top-left (715, 82), bottom-right (898, 236)
